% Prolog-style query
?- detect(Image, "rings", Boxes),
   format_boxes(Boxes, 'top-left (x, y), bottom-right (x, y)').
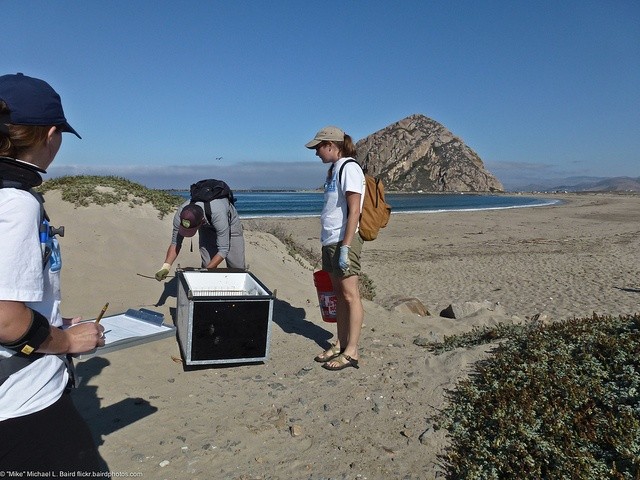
top-left (100, 331), bottom-right (104, 338)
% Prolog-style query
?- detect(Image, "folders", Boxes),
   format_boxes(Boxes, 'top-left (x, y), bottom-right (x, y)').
top-left (61, 307), bottom-right (177, 359)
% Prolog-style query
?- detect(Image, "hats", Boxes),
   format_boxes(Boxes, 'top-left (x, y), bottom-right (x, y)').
top-left (179, 205), bottom-right (205, 237)
top-left (304, 126), bottom-right (344, 149)
top-left (1, 72), bottom-right (81, 140)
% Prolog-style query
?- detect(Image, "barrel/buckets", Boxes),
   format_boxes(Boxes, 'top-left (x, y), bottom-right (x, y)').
top-left (313, 270), bottom-right (338, 322)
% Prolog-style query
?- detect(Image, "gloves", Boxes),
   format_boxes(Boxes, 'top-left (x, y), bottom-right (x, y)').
top-left (339, 245), bottom-right (351, 271)
top-left (155, 263), bottom-right (171, 281)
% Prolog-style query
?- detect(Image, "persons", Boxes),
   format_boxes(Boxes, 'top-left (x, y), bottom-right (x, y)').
top-left (1, 72), bottom-right (110, 478)
top-left (305, 127), bottom-right (367, 371)
top-left (155, 197), bottom-right (245, 281)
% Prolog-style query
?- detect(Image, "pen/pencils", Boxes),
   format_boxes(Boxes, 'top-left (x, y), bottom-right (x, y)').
top-left (95, 303), bottom-right (109, 325)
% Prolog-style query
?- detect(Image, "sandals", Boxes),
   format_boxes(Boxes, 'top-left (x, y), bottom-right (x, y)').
top-left (323, 353), bottom-right (359, 370)
top-left (314, 343), bottom-right (346, 363)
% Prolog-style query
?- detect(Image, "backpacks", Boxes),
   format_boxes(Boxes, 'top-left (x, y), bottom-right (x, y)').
top-left (189, 179), bottom-right (234, 224)
top-left (338, 159), bottom-right (392, 241)
